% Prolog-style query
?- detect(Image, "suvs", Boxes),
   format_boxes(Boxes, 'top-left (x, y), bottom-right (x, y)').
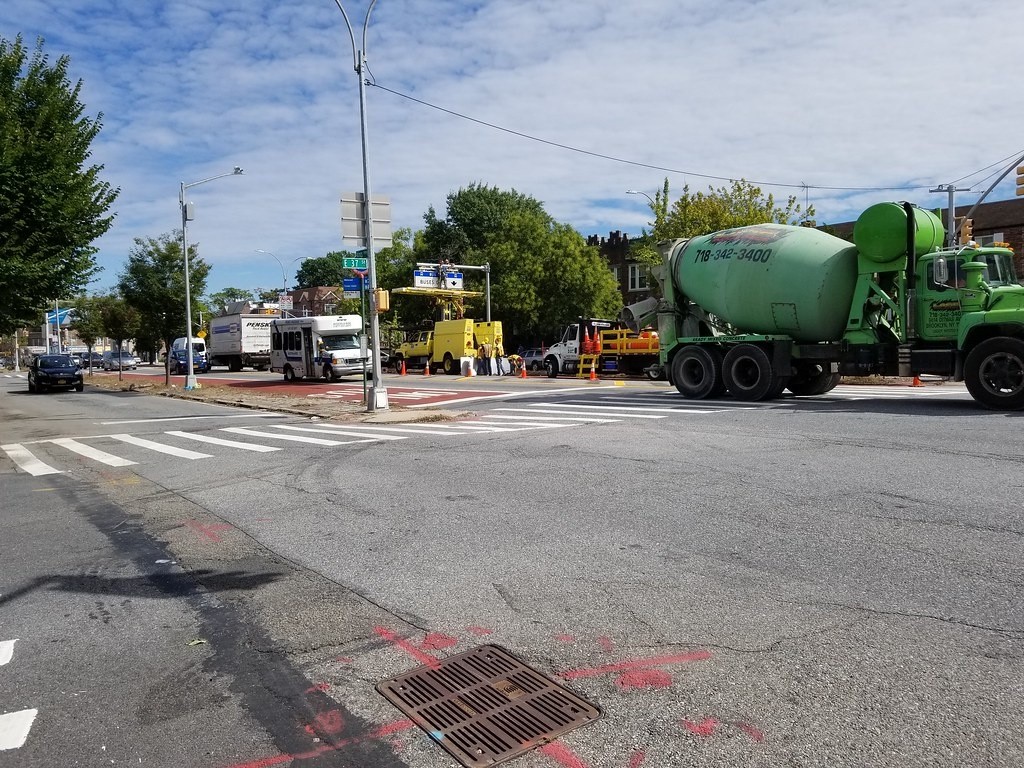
top-left (516, 347), bottom-right (550, 372)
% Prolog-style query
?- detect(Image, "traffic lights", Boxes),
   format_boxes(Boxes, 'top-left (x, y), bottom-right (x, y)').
top-left (270, 308), bottom-right (274, 314)
top-left (265, 309), bottom-right (270, 313)
top-left (961, 217), bottom-right (975, 244)
top-left (1015, 165), bottom-right (1024, 196)
top-left (202, 320), bottom-right (207, 329)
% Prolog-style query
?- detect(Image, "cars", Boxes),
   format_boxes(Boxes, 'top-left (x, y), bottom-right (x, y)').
top-left (380, 349), bottom-right (396, 368)
top-left (28, 354), bottom-right (84, 393)
top-left (165, 348), bottom-right (208, 375)
top-left (0, 349), bottom-right (142, 371)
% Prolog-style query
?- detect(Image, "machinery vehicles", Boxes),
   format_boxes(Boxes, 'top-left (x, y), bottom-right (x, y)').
top-left (651, 204), bottom-right (1024, 412)
top-left (391, 287), bottom-right (504, 375)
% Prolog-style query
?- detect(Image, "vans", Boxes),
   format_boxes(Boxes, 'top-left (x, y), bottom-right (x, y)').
top-left (270, 315), bottom-right (372, 383)
top-left (169, 337), bottom-right (207, 364)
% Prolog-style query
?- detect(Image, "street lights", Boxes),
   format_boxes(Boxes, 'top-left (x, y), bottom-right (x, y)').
top-left (256, 248), bottom-right (316, 317)
top-left (178, 166), bottom-right (245, 390)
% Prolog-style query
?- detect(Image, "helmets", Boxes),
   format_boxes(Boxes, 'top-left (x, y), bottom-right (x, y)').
top-left (485, 337), bottom-right (489, 343)
top-left (481, 341), bottom-right (485, 344)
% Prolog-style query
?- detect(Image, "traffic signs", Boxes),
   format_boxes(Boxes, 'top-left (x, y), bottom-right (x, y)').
top-left (413, 270), bottom-right (464, 291)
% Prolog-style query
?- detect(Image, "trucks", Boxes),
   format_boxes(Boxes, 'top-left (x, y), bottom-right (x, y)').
top-left (542, 323), bottom-right (667, 381)
top-left (206, 314), bottom-right (281, 372)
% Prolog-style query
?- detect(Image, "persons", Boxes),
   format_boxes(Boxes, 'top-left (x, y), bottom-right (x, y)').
top-left (445, 258), bottom-right (459, 273)
top-left (478, 339), bottom-right (504, 375)
top-left (948, 264), bottom-right (966, 287)
top-left (507, 354), bottom-right (523, 374)
top-left (435, 259), bottom-right (448, 290)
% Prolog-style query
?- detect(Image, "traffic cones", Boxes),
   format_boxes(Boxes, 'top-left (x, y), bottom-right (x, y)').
top-left (423, 360), bottom-right (432, 376)
top-left (581, 324), bottom-right (601, 355)
top-left (466, 360), bottom-right (474, 377)
top-left (519, 359), bottom-right (529, 378)
top-left (400, 360), bottom-right (407, 376)
top-left (589, 359), bottom-right (597, 380)
top-left (908, 374), bottom-right (926, 387)
